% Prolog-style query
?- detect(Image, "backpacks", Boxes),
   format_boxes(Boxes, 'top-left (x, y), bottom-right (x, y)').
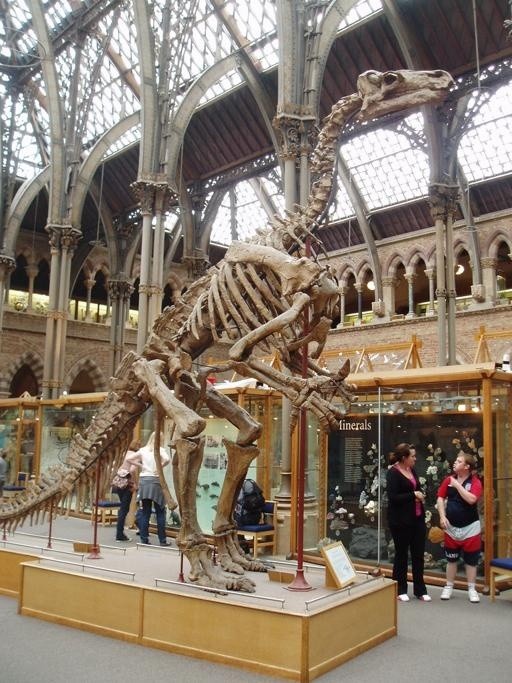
top-left (231, 477), bottom-right (266, 528)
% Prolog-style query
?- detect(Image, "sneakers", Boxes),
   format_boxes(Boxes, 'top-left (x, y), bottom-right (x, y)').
top-left (398, 593), bottom-right (410, 602)
top-left (115, 529), bottom-right (172, 546)
top-left (466, 588), bottom-right (480, 603)
top-left (416, 593), bottom-right (432, 602)
top-left (439, 585), bottom-right (454, 600)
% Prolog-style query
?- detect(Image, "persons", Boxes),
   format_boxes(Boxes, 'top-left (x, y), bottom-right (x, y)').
top-left (0, 448), bottom-right (9, 509)
top-left (385, 443), bottom-right (432, 603)
top-left (233, 478), bottom-right (265, 554)
top-left (330, 303), bottom-right (341, 329)
top-left (436, 454), bottom-right (483, 603)
top-left (126, 430), bottom-right (173, 546)
top-left (107, 439), bottom-right (142, 540)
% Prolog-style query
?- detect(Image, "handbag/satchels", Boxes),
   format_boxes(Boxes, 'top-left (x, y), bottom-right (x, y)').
top-left (110, 468), bottom-right (131, 490)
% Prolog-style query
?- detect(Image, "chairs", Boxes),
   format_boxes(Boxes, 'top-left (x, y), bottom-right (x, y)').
top-left (3, 472), bottom-right (29, 502)
top-left (234, 500), bottom-right (277, 559)
top-left (90, 485), bottom-right (122, 527)
top-left (490, 557), bottom-right (512, 599)
